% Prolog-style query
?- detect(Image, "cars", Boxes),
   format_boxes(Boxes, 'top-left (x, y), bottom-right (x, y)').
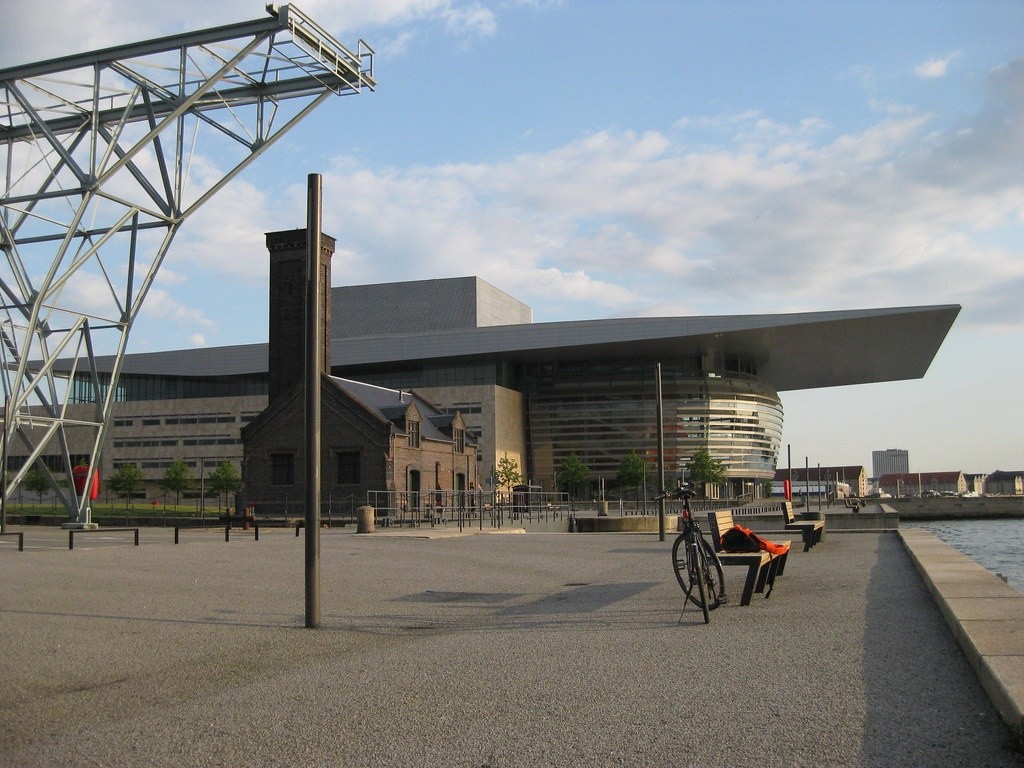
top-left (880, 494), bottom-right (891, 498)
top-left (849, 493), bottom-right (856, 498)
top-left (870, 491), bottom-right (883, 498)
top-left (861, 495), bottom-right (866, 498)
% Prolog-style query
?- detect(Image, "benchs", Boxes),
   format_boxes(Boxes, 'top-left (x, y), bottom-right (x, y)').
top-left (484, 503), bottom-right (493, 518)
top-left (845, 499), bottom-right (867, 513)
top-left (547, 503), bottom-right (560, 511)
top-left (705, 511), bottom-right (791, 606)
top-left (781, 502), bottom-right (825, 552)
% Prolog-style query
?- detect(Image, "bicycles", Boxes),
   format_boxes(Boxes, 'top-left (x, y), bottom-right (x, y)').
top-left (652, 478), bottom-right (731, 625)
